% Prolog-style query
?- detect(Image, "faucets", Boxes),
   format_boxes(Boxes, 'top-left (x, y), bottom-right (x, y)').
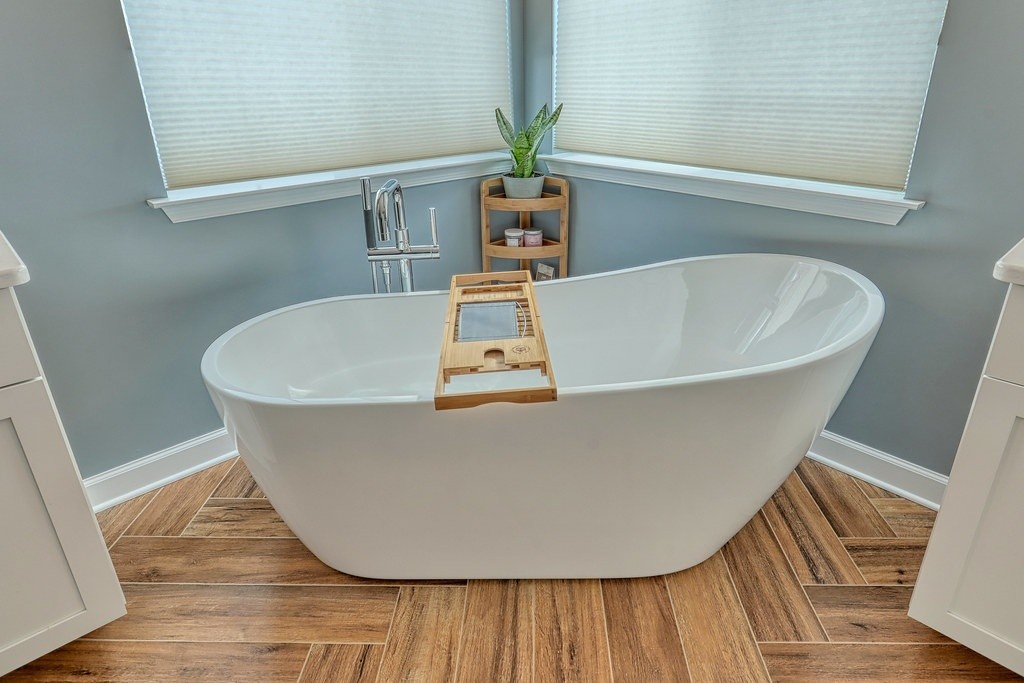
top-left (373, 178), bottom-right (411, 248)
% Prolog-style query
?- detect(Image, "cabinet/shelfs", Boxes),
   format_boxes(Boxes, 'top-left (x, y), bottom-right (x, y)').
top-left (907, 238), bottom-right (1024, 680)
top-left (0, 229), bottom-right (129, 678)
top-left (479, 177), bottom-right (570, 285)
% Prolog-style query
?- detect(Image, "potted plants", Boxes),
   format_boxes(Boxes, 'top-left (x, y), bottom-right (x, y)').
top-left (495, 103), bottom-right (563, 198)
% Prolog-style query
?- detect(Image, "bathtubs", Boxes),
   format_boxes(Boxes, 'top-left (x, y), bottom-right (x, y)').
top-left (200, 252), bottom-right (885, 582)
